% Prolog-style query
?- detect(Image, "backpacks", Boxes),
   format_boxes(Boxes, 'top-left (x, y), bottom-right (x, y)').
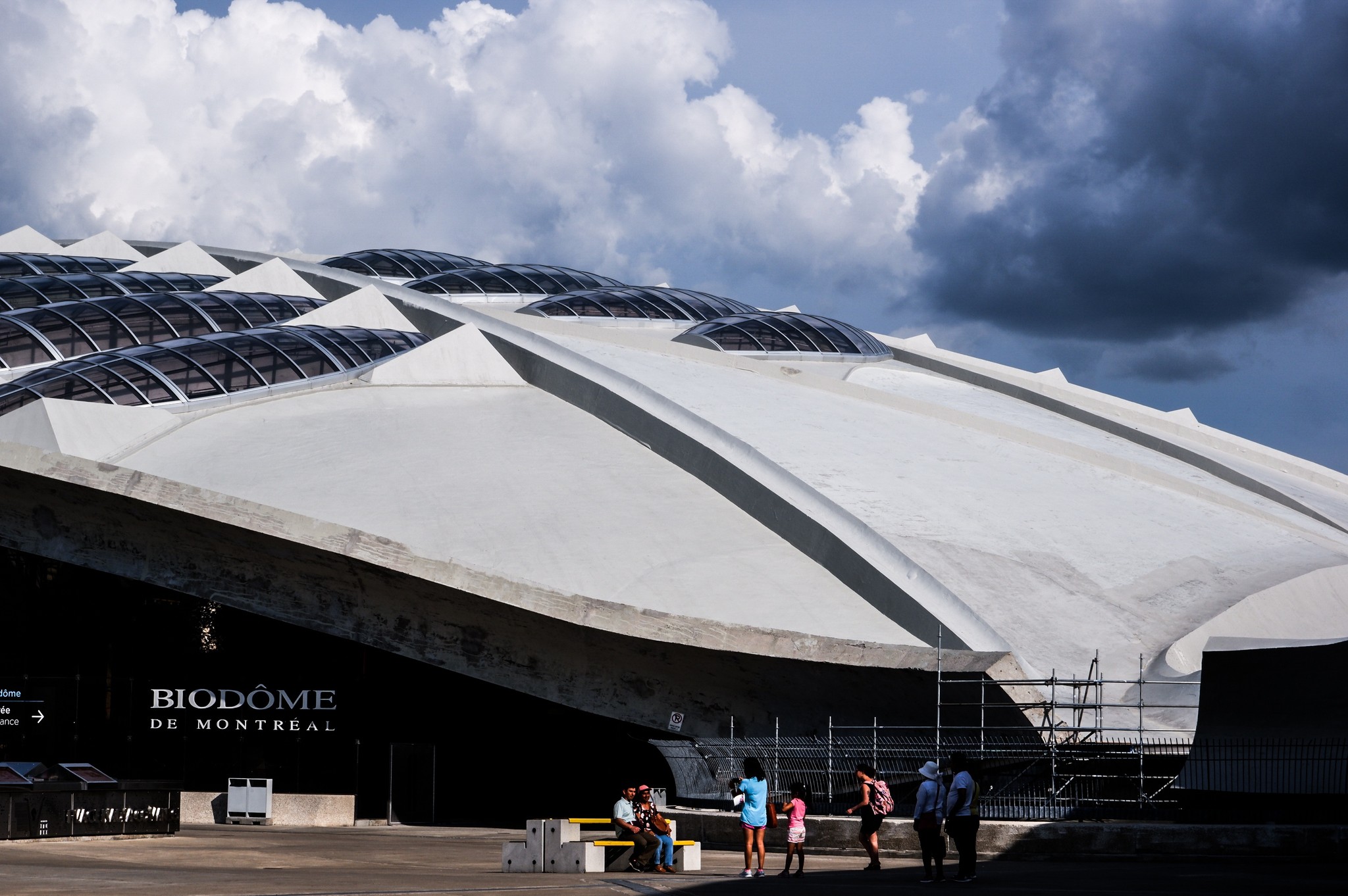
top-left (859, 779), bottom-right (894, 816)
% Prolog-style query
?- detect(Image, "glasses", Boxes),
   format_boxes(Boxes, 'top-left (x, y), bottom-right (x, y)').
top-left (855, 770), bottom-right (859, 773)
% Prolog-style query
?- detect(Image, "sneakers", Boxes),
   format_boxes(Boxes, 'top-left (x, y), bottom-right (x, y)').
top-left (793, 870), bottom-right (805, 878)
top-left (778, 870), bottom-right (790, 877)
top-left (754, 869), bottom-right (764, 877)
top-left (739, 868), bottom-right (752, 878)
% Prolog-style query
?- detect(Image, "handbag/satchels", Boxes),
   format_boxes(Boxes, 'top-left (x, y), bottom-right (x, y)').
top-left (766, 802), bottom-right (777, 829)
top-left (939, 835), bottom-right (946, 858)
top-left (648, 802), bottom-right (672, 835)
top-left (913, 810), bottom-right (936, 831)
top-left (627, 821), bottom-right (644, 833)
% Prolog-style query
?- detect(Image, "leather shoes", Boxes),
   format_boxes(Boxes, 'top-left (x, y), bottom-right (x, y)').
top-left (628, 858), bottom-right (644, 872)
top-left (662, 865), bottom-right (676, 873)
top-left (654, 864), bottom-right (665, 873)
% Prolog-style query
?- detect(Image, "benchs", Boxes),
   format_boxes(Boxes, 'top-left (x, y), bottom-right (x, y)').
top-left (502, 818), bottom-right (702, 873)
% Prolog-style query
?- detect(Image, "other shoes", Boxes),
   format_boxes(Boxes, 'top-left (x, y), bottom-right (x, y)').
top-left (917, 876), bottom-right (935, 882)
top-left (935, 875), bottom-right (946, 882)
top-left (950, 874), bottom-right (972, 882)
top-left (970, 870), bottom-right (979, 878)
top-left (864, 862), bottom-right (881, 870)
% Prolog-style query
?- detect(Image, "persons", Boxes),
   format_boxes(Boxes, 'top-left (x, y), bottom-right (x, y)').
top-left (913, 761), bottom-right (946, 883)
top-left (846, 764), bottom-right (883, 871)
top-left (613, 784), bottom-right (676, 872)
top-left (944, 754), bottom-right (980, 882)
top-left (728, 757), bottom-right (768, 877)
top-left (778, 782), bottom-right (807, 875)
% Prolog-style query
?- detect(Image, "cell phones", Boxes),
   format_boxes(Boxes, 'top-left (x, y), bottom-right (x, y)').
top-left (733, 777), bottom-right (741, 783)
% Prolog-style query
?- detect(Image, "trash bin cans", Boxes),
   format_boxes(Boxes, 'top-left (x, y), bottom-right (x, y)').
top-left (648, 788), bottom-right (666, 806)
top-left (225, 778), bottom-right (273, 826)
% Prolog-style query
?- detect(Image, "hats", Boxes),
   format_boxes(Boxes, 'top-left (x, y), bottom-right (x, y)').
top-left (919, 761), bottom-right (944, 786)
top-left (638, 785), bottom-right (652, 793)
